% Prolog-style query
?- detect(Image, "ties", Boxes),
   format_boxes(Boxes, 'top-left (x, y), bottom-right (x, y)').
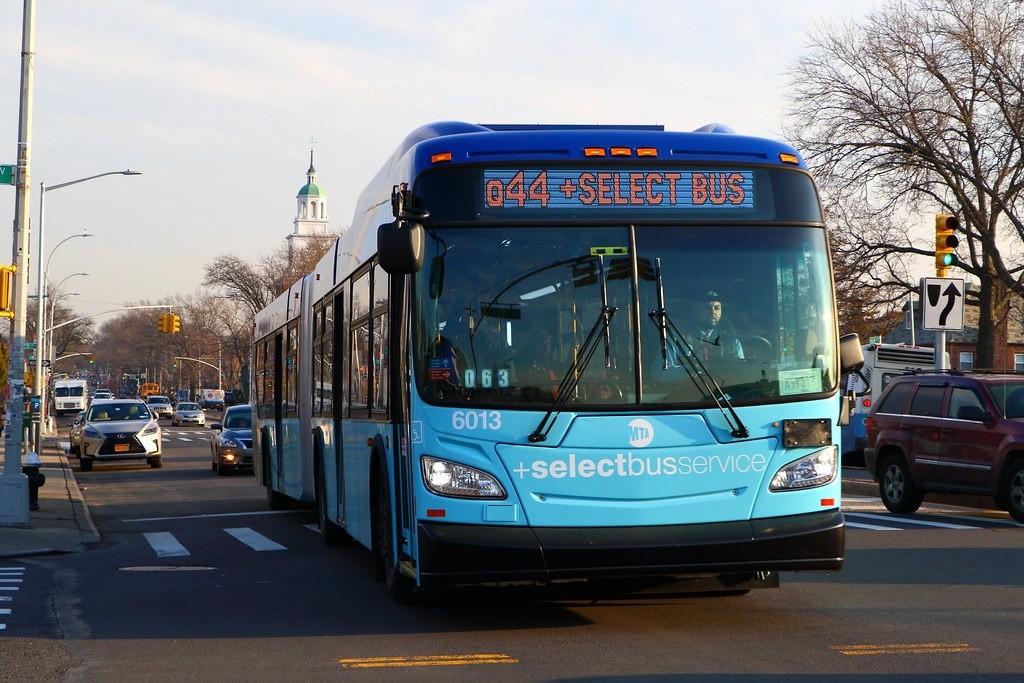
top-left (702, 335), bottom-right (708, 361)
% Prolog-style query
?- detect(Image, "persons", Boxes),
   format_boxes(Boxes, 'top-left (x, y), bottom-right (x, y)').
top-left (649, 285), bottom-right (747, 385)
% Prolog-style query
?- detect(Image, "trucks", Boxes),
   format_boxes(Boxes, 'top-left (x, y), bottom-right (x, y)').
top-left (198, 389), bottom-right (225, 412)
top-left (53, 378), bottom-right (87, 414)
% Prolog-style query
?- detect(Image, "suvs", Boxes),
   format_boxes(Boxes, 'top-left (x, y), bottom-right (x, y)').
top-left (866, 370), bottom-right (1024, 524)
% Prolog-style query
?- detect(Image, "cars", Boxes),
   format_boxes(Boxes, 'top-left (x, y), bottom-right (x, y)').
top-left (172, 403), bottom-right (205, 426)
top-left (211, 403), bottom-right (254, 474)
top-left (74, 398), bottom-right (163, 469)
top-left (91, 389), bottom-right (114, 400)
top-left (146, 394), bottom-right (171, 420)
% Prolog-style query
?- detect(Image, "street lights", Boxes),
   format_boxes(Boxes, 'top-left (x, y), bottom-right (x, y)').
top-left (200, 326), bottom-right (225, 388)
top-left (48, 270), bottom-right (92, 419)
top-left (44, 231), bottom-right (92, 431)
top-left (33, 170), bottom-right (145, 433)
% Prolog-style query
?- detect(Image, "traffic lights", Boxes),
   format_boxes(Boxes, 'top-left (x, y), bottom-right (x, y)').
top-left (169, 315), bottom-right (180, 334)
top-left (158, 313), bottom-right (169, 333)
top-left (935, 212), bottom-right (959, 274)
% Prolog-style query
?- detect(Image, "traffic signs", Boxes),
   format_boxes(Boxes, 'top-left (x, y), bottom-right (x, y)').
top-left (921, 277), bottom-right (965, 330)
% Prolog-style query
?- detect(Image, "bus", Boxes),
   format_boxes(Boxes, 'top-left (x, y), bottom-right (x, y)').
top-left (838, 343), bottom-right (955, 466)
top-left (233, 119), bottom-right (872, 599)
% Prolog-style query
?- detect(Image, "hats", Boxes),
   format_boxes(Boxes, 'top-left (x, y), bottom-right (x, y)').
top-left (692, 288), bottom-right (723, 302)
top-left (517, 328), bottom-right (552, 347)
